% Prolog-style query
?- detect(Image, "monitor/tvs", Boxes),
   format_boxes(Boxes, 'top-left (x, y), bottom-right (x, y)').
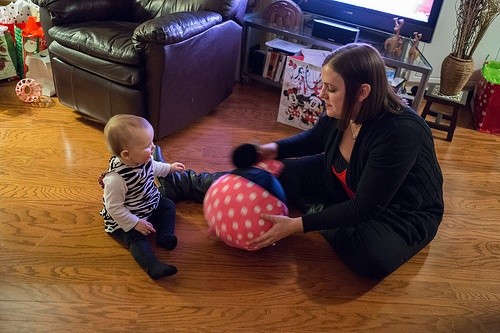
top-left (294, 0), bottom-right (444, 43)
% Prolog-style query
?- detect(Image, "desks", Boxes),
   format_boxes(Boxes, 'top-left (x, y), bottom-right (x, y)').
top-left (421, 84), bottom-right (469, 141)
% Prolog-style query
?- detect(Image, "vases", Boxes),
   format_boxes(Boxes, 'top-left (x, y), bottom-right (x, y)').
top-left (440, 53), bottom-right (475, 96)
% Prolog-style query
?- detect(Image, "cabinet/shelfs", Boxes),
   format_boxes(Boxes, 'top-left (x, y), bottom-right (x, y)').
top-left (242, 9), bottom-right (433, 111)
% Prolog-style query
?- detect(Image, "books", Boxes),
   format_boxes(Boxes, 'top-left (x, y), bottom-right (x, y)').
top-left (262, 51), bottom-right (290, 83)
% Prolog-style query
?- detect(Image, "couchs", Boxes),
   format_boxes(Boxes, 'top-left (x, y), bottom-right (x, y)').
top-left (32, 0), bottom-right (254, 142)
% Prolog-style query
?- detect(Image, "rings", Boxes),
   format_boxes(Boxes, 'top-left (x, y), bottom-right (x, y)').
top-left (272, 242), bottom-right (276, 246)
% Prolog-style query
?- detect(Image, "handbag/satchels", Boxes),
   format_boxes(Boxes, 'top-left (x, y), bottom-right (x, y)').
top-left (276, 56), bottom-right (326, 132)
top-left (473, 54), bottom-right (500, 135)
top-left (0, 15), bottom-right (47, 84)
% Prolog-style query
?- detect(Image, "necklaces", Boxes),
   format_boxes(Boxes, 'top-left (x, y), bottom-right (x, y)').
top-left (350, 121), bottom-right (362, 140)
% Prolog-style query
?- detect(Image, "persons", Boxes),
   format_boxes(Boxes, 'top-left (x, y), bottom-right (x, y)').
top-left (99, 114), bottom-right (185, 281)
top-left (153, 43), bottom-right (444, 280)
